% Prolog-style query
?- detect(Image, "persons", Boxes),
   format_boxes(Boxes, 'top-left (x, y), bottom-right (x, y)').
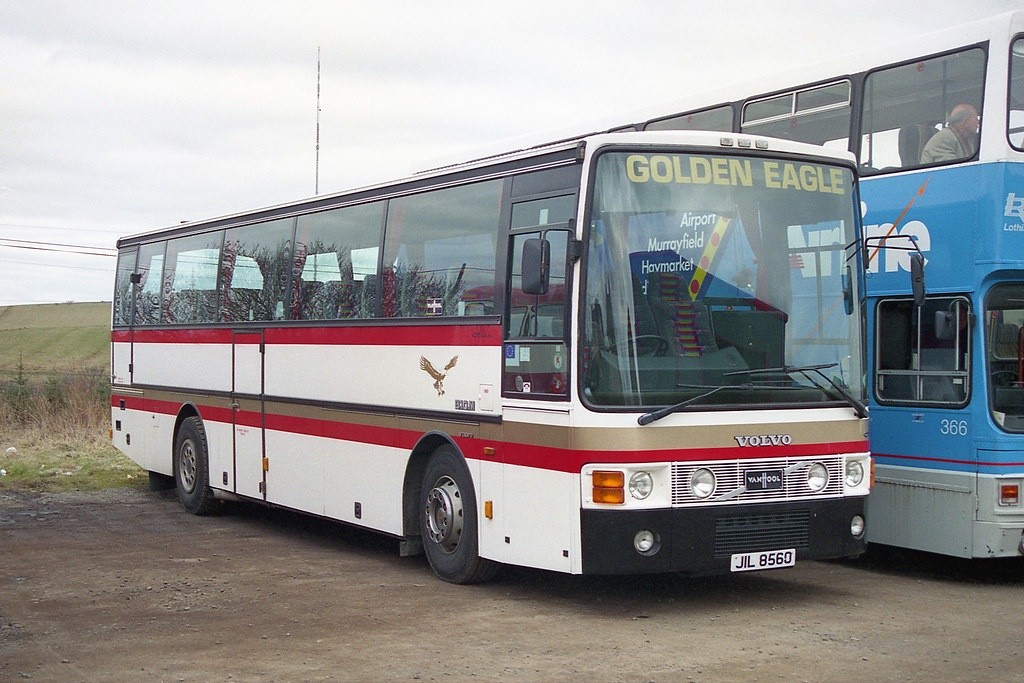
top-left (938, 300), bottom-right (971, 373)
top-left (919, 103), bottom-right (980, 166)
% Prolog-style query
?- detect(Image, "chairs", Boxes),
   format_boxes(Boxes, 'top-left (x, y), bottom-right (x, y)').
top-left (647, 261), bottom-right (720, 357)
top-left (119, 265), bottom-right (480, 324)
top-left (606, 275), bottom-right (664, 359)
top-left (996, 322), bottom-right (1024, 358)
top-left (898, 124), bottom-right (941, 166)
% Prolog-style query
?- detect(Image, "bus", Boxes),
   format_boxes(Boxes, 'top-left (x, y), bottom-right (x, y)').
top-left (426, 9), bottom-right (1024, 559)
top-left (105, 132), bottom-right (875, 587)
